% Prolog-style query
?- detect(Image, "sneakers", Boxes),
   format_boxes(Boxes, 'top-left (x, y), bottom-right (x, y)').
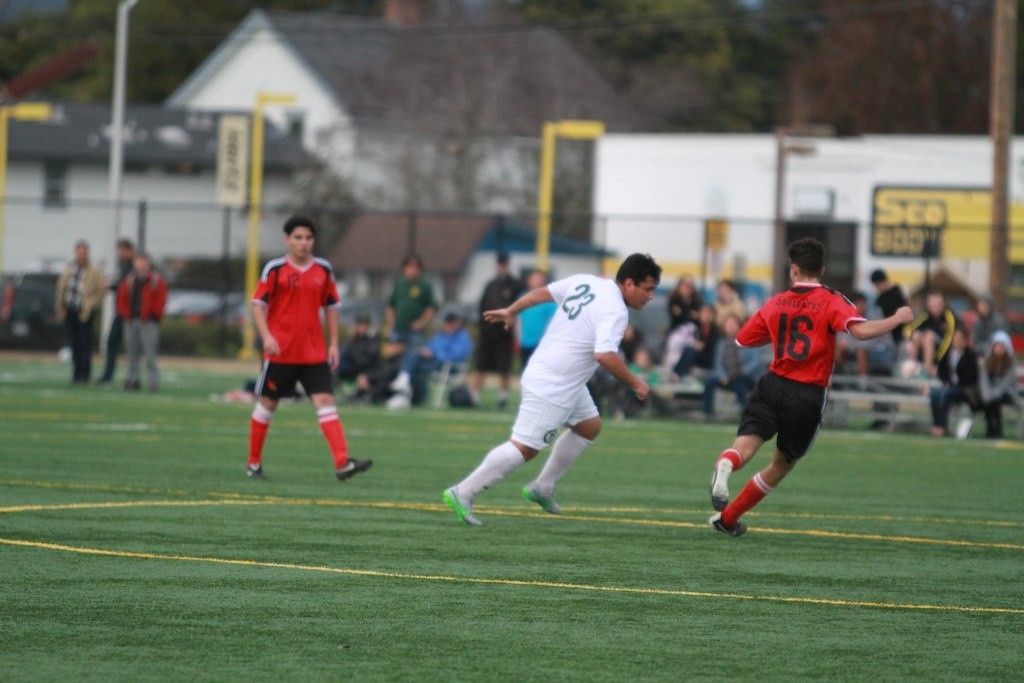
top-left (710, 458), bottom-right (733, 511)
top-left (709, 510), bottom-right (748, 537)
top-left (443, 485), bottom-right (482, 527)
top-left (522, 481), bottom-right (561, 515)
top-left (247, 466), bottom-right (265, 480)
top-left (336, 458), bottom-right (373, 482)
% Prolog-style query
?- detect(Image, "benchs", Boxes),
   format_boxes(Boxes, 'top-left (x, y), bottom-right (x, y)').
top-left (681, 367), bottom-right (1024, 439)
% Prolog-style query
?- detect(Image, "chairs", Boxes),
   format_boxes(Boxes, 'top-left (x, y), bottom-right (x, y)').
top-left (426, 361), bottom-right (471, 405)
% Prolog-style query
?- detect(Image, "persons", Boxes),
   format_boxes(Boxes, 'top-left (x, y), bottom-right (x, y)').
top-left (244, 217), bottom-right (374, 482)
top-left (709, 239), bottom-right (915, 538)
top-left (442, 253), bottom-right (664, 525)
top-left (1, 238), bottom-right (1023, 440)
